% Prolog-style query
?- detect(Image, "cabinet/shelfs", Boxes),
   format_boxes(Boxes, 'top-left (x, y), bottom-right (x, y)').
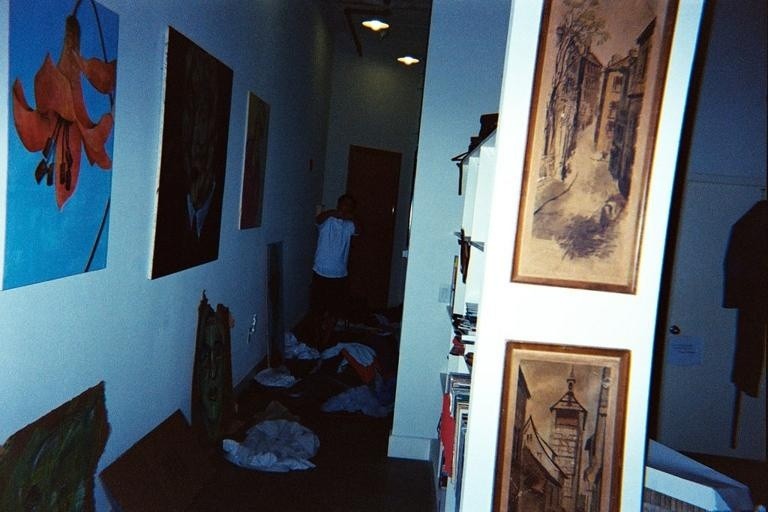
top-left (428, 110), bottom-right (692, 512)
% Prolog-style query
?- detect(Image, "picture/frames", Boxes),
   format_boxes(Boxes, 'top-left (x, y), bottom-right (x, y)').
top-left (509, 1), bottom-right (682, 296)
top-left (488, 340), bottom-right (633, 512)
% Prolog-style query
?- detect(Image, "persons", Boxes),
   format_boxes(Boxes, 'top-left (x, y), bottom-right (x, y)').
top-left (308, 193), bottom-right (362, 350)
